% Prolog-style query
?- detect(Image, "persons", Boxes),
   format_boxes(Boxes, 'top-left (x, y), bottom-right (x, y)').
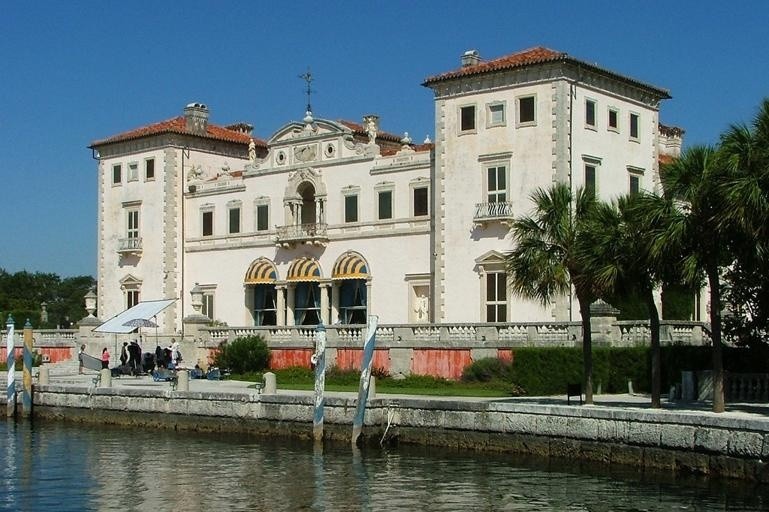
top-left (101, 348), bottom-right (110, 369)
top-left (78, 344), bottom-right (87, 375)
top-left (365, 117), bottom-right (378, 143)
top-left (119, 337), bottom-right (180, 376)
top-left (248, 138), bottom-right (256, 160)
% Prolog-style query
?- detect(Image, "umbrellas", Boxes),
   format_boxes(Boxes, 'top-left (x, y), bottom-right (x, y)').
top-left (122, 318), bottom-right (160, 345)
